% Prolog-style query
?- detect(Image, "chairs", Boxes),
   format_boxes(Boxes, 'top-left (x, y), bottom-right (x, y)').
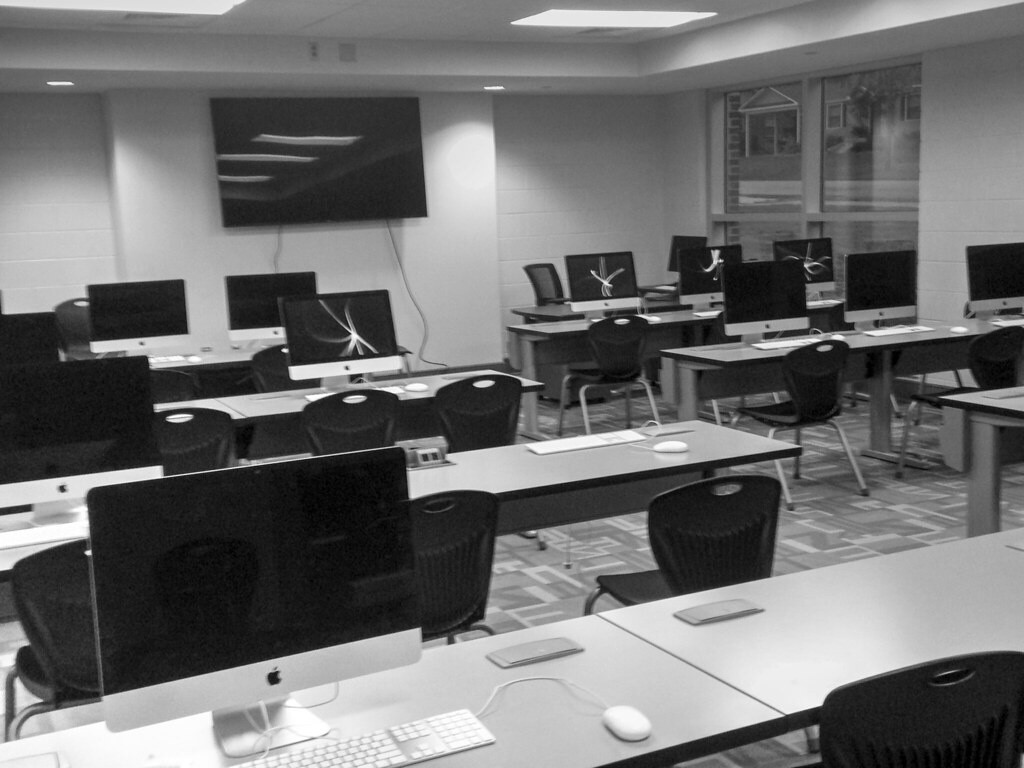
top-left (800, 647), bottom-right (1024, 768)
top-left (3, 536), bottom-right (103, 735)
top-left (726, 340), bottom-right (870, 511)
top-left (556, 315), bottom-right (662, 437)
top-left (585, 475), bottom-right (782, 615)
top-left (433, 373), bottom-right (544, 549)
top-left (249, 342), bottom-right (363, 394)
top-left (405, 490), bottom-right (500, 649)
top-left (828, 301), bottom-right (886, 331)
top-left (895, 324), bottom-right (1024, 480)
top-left (524, 264), bottom-right (574, 306)
top-left (150, 368), bottom-right (201, 407)
top-left (301, 388), bottom-right (401, 455)
top-left (57, 296), bottom-right (124, 361)
top-left (153, 407), bottom-right (237, 478)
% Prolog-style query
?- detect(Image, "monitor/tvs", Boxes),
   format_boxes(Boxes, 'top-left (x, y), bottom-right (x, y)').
top-left (564, 250), bottom-right (641, 319)
top-left (966, 242), bottom-right (1024, 321)
top-left (0, 271), bottom-right (403, 529)
top-left (211, 96), bottom-right (427, 226)
top-left (88, 446), bottom-right (422, 759)
top-left (678, 237), bottom-right (917, 344)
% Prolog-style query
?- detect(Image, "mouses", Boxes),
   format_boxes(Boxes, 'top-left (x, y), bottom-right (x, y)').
top-left (653, 440), bottom-right (688, 453)
top-left (650, 316), bottom-right (661, 322)
top-left (831, 334), bottom-right (845, 341)
top-left (406, 382), bottom-right (428, 391)
top-left (951, 327), bottom-right (967, 334)
top-left (603, 705), bottom-right (652, 742)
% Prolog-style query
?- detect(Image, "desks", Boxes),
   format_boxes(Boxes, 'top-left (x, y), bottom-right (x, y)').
top-left (397, 418), bottom-right (803, 539)
top-left (57, 325), bottom-right (288, 376)
top-left (0, 613), bottom-right (788, 768)
top-left (510, 297), bottom-right (846, 438)
top-left (514, 297), bottom-right (692, 320)
top-left (598, 528), bottom-right (1024, 731)
top-left (156, 397), bottom-right (250, 463)
top-left (219, 367), bottom-right (544, 460)
top-left (934, 383), bottom-right (1024, 539)
top-left (658, 314), bottom-right (1024, 476)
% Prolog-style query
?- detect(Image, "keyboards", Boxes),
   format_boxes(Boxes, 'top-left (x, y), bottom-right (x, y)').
top-left (863, 326), bottom-right (933, 337)
top-left (225, 710), bottom-right (494, 768)
top-left (806, 299), bottom-right (842, 306)
top-left (0, 521), bottom-right (88, 549)
top-left (991, 319), bottom-right (1024, 327)
top-left (525, 428), bottom-right (646, 456)
top-left (592, 314), bottom-right (650, 323)
top-left (694, 310), bottom-right (722, 317)
top-left (751, 339), bottom-right (821, 350)
top-left (306, 386), bottom-right (404, 401)
